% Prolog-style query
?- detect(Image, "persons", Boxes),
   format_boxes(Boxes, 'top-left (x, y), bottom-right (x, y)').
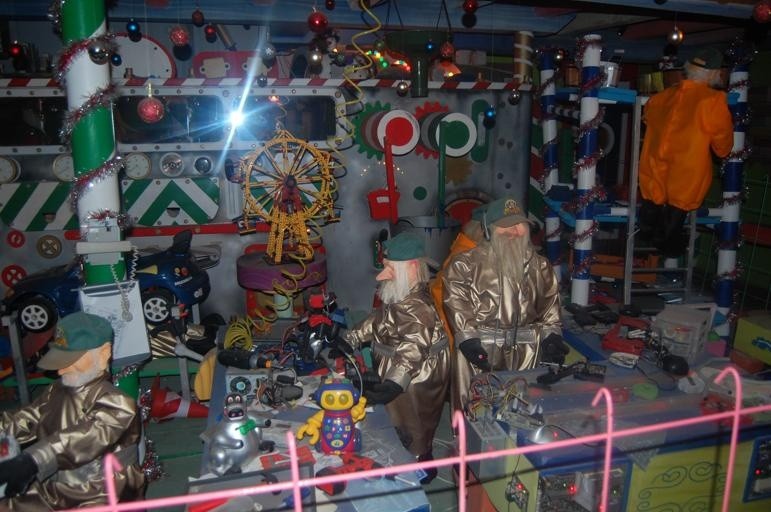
top-left (2, 312), bottom-right (141, 511)
top-left (328, 230), bottom-right (451, 487)
top-left (638, 44), bottom-right (734, 257)
top-left (430, 186), bottom-right (569, 418)
